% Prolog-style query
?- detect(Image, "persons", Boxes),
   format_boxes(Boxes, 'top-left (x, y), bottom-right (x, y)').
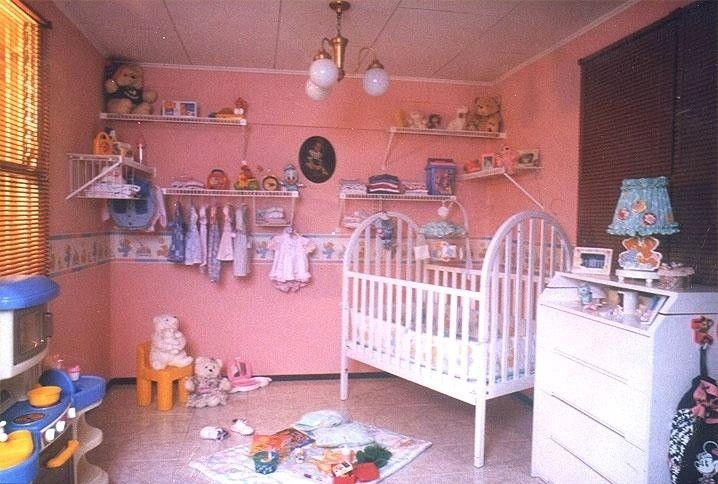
top-left (304, 139), bottom-right (331, 178)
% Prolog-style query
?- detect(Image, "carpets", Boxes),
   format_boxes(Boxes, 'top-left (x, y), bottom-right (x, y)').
top-left (187, 409), bottom-right (436, 483)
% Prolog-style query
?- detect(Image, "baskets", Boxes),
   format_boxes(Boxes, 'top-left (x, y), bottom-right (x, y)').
top-left (252, 445), bottom-right (278, 474)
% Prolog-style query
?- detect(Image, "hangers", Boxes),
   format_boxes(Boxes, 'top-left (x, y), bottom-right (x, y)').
top-left (174, 194), bottom-right (245, 208)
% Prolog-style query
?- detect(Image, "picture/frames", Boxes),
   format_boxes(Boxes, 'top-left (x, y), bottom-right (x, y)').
top-left (516, 148), bottom-right (539, 167)
top-left (573, 245), bottom-right (612, 279)
top-left (298, 136), bottom-right (337, 184)
top-left (161, 99), bottom-right (198, 118)
top-left (480, 152), bottom-right (494, 170)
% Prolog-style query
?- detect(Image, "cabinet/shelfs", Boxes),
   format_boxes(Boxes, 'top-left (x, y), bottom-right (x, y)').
top-left (158, 185), bottom-right (300, 229)
top-left (66, 153), bottom-right (155, 202)
top-left (530, 271), bottom-right (717, 484)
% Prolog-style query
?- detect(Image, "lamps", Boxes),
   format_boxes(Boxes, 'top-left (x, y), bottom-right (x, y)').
top-left (606, 175), bottom-right (681, 272)
top-left (304, 1), bottom-right (392, 102)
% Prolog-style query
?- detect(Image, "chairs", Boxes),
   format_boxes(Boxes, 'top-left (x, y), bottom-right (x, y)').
top-left (136, 337), bottom-right (195, 410)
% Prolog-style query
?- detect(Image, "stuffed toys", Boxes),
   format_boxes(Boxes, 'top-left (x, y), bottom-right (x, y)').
top-left (104, 61), bottom-right (157, 116)
top-left (150, 312), bottom-right (193, 370)
top-left (185, 357), bottom-right (233, 408)
top-left (471, 92), bottom-right (503, 132)
top-left (283, 163), bottom-right (302, 192)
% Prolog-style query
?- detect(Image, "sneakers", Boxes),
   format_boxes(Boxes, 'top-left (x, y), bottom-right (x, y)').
top-left (200, 426), bottom-right (230, 442)
top-left (231, 419), bottom-right (254, 435)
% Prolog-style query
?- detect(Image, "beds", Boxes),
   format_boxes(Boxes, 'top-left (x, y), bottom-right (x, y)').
top-left (337, 210), bottom-right (573, 467)
